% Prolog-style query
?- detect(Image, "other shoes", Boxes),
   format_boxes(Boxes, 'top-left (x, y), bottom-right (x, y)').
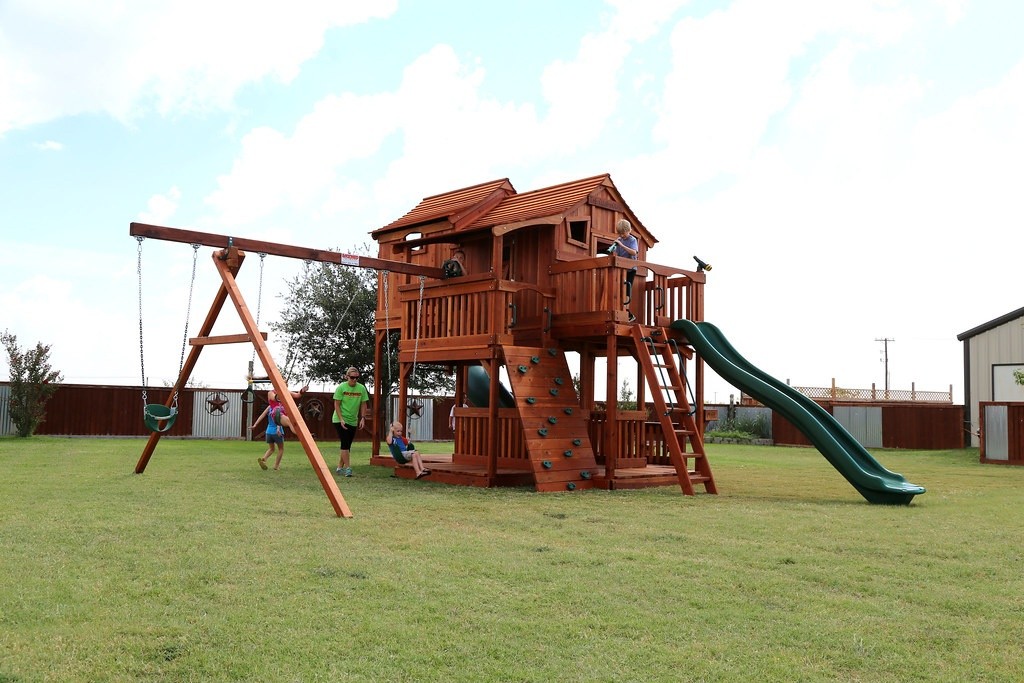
top-left (257, 458), bottom-right (268, 470)
top-left (336, 468), bottom-right (344, 475)
top-left (345, 467), bottom-right (352, 477)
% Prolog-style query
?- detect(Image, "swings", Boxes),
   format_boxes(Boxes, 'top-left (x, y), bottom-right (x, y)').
top-left (248, 252), bottom-right (309, 384)
top-left (138, 252), bottom-right (197, 433)
top-left (383, 275), bottom-right (424, 464)
top-left (273, 268), bottom-right (368, 427)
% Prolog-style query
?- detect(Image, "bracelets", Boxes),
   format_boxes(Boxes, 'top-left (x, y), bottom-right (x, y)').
top-left (300, 389), bottom-right (306, 395)
top-left (362, 416), bottom-right (365, 419)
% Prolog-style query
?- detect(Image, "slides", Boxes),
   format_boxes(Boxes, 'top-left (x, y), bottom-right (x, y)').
top-left (671, 318), bottom-right (926, 505)
top-left (467, 366), bottom-right (514, 407)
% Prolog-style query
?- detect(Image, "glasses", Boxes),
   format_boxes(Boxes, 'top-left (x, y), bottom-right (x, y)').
top-left (349, 375), bottom-right (359, 380)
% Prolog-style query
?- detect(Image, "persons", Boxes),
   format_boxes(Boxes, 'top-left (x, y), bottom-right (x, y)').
top-left (332, 367), bottom-right (369, 477)
top-left (613, 219), bottom-right (639, 323)
top-left (449, 392), bottom-right (471, 438)
top-left (452, 250), bottom-right (468, 311)
top-left (386, 421), bottom-right (432, 480)
top-left (248, 375), bottom-right (314, 470)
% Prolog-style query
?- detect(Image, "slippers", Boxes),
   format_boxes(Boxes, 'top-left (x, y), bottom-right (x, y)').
top-left (423, 469), bottom-right (431, 474)
top-left (415, 472), bottom-right (428, 479)
